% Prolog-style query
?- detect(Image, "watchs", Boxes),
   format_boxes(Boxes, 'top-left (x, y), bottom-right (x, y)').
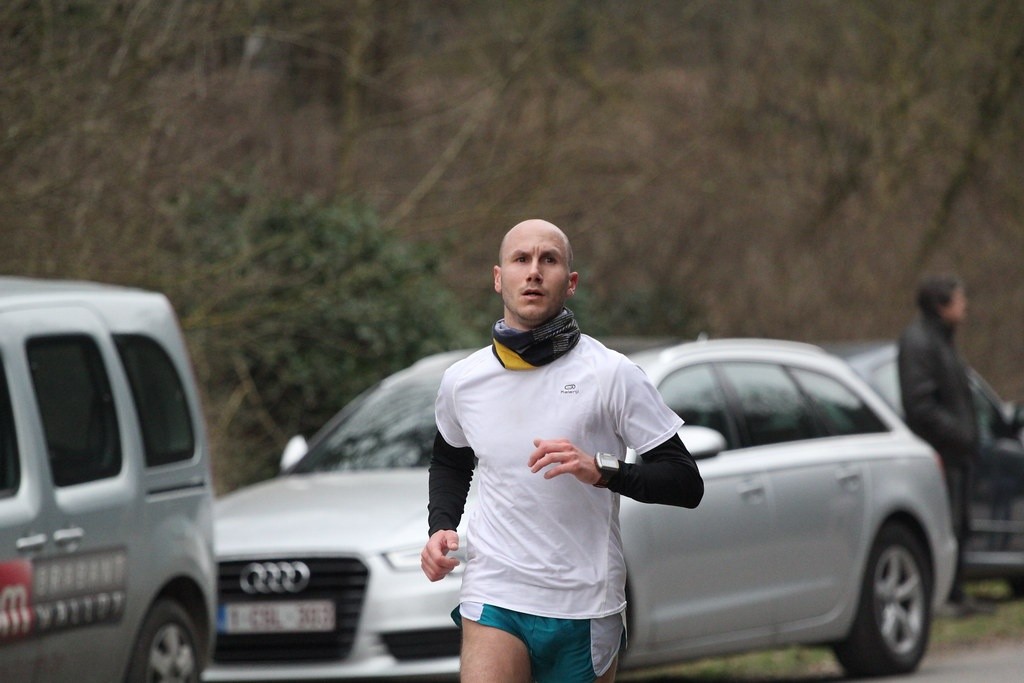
top-left (594, 452), bottom-right (619, 488)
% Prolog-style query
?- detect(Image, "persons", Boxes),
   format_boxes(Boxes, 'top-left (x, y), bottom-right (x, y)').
top-left (896, 267), bottom-right (1024, 608)
top-left (422, 218), bottom-right (705, 683)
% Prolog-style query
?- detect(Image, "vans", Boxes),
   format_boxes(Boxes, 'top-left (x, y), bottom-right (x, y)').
top-left (0, 270), bottom-right (225, 683)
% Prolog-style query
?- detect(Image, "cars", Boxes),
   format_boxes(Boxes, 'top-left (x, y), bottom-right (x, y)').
top-left (196, 327), bottom-right (967, 683)
top-left (824, 338), bottom-right (1024, 603)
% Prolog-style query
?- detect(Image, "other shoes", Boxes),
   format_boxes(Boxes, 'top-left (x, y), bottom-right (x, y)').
top-left (943, 597), bottom-right (992, 618)
